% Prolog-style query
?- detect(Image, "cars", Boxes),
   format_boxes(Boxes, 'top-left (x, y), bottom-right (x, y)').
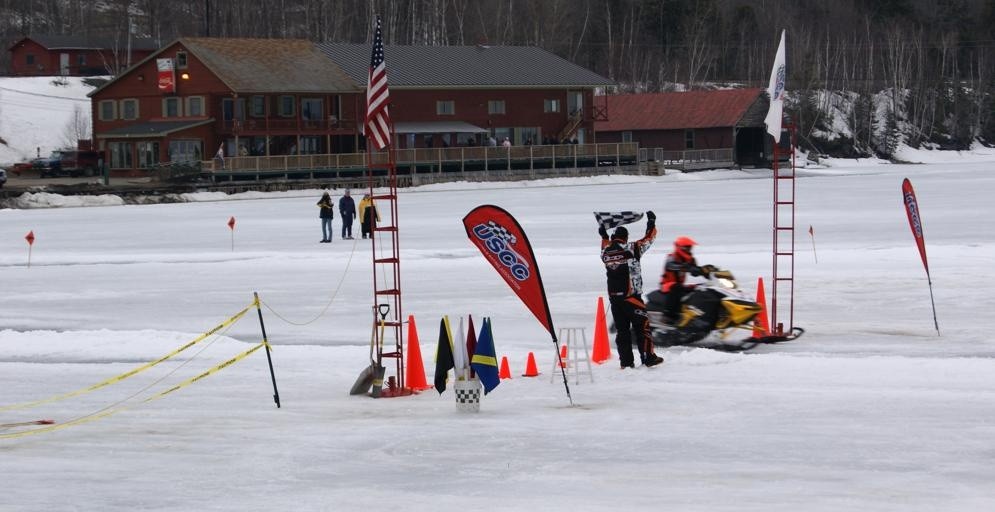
top-left (11, 156), bottom-right (50, 177)
top-left (0, 167), bottom-right (7, 188)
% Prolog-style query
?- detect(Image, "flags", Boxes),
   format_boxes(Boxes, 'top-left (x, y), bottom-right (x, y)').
top-left (359, 14), bottom-right (395, 152)
top-left (762, 27), bottom-right (792, 145)
top-left (215, 141), bottom-right (227, 169)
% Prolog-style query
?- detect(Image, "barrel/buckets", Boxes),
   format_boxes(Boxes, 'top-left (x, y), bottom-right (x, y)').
top-left (453, 378), bottom-right (483, 414)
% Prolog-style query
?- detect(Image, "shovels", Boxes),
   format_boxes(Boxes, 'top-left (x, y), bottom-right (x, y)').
top-left (350, 304), bottom-right (390, 398)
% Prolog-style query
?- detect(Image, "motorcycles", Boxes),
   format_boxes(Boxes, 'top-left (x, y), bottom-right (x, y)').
top-left (612, 262), bottom-right (805, 355)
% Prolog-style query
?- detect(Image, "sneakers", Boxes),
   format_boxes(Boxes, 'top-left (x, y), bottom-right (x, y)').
top-left (620, 362), bottom-right (635, 369)
top-left (644, 356), bottom-right (665, 368)
top-left (320, 236), bottom-right (373, 242)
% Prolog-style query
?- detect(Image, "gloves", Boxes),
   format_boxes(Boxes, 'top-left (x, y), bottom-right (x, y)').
top-left (647, 210), bottom-right (657, 222)
top-left (598, 225), bottom-right (609, 237)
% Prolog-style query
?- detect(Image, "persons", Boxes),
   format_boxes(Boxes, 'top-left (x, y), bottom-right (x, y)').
top-left (338, 187), bottom-right (356, 240)
top-left (596, 210), bottom-right (664, 371)
top-left (315, 191), bottom-right (334, 243)
top-left (466, 134), bottom-right (578, 147)
top-left (358, 191), bottom-right (381, 240)
top-left (655, 236), bottom-right (718, 323)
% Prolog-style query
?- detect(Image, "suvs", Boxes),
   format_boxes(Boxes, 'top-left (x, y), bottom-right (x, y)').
top-left (33, 150), bottom-right (99, 177)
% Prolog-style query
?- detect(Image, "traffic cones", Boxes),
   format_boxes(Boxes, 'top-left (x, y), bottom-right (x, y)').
top-left (553, 345), bottom-right (569, 370)
top-left (499, 357), bottom-right (512, 382)
top-left (519, 349), bottom-right (540, 378)
top-left (405, 314), bottom-right (434, 393)
top-left (591, 296), bottom-right (615, 368)
top-left (750, 278), bottom-right (775, 343)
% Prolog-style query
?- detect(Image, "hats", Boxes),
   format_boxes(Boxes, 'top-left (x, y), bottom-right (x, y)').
top-left (675, 237), bottom-right (698, 247)
top-left (611, 227), bottom-right (628, 237)
